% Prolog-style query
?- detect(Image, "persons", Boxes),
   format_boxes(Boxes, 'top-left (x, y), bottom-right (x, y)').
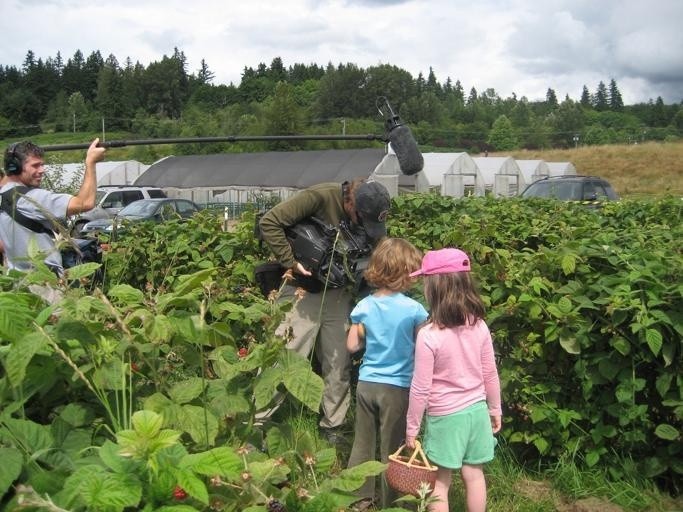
top-left (338, 232), bottom-right (438, 511)
top-left (234, 179), bottom-right (391, 454)
top-left (399, 246), bottom-right (503, 511)
top-left (0, 132), bottom-right (106, 327)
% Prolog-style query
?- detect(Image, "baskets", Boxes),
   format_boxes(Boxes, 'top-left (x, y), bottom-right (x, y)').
top-left (387, 439), bottom-right (438, 499)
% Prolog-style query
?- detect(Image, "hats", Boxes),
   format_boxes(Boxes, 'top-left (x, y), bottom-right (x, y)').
top-left (410, 248), bottom-right (471, 276)
top-left (353, 181), bottom-right (391, 239)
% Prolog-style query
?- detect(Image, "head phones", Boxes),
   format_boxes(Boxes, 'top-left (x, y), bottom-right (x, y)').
top-left (4, 142), bottom-right (23, 175)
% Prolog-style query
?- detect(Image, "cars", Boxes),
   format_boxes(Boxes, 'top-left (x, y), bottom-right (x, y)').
top-left (78, 197), bottom-right (201, 238)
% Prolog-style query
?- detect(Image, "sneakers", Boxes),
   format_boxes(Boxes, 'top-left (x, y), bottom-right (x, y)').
top-left (320, 428), bottom-right (346, 450)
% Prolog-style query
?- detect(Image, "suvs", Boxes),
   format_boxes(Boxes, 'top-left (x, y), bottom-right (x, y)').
top-left (515, 174), bottom-right (617, 209)
top-left (64, 186), bottom-right (169, 238)
